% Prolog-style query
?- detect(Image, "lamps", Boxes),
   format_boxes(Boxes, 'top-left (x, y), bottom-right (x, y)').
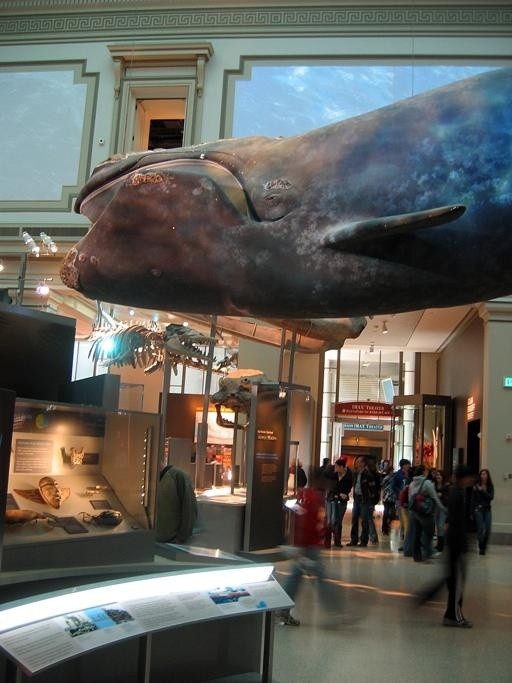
top-left (21, 229), bottom-right (58, 256)
top-left (0, 562), bottom-right (277, 634)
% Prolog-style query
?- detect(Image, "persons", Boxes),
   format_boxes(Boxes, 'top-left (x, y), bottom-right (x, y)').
top-left (275, 467), bottom-right (363, 629)
top-left (286, 452), bottom-right (496, 564)
top-left (154, 449), bottom-right (198, 545)
top-left (209, 454), bottom-right (216, 463)
top-left (220, 465), bottom-right (232, 486)
top-left (406, 463), bottom-right (479, 629)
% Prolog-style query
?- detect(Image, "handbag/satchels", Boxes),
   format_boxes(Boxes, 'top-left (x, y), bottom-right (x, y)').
top-left (410, 493), bottom-right (435, 516)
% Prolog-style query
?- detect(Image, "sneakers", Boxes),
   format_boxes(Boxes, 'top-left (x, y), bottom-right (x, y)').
top-left (443, 618), bottom-right (472, 628)
top-left (279, 615), bottom-right (300, 626)
top-left (324, 541), bottom-right (378, 548)
